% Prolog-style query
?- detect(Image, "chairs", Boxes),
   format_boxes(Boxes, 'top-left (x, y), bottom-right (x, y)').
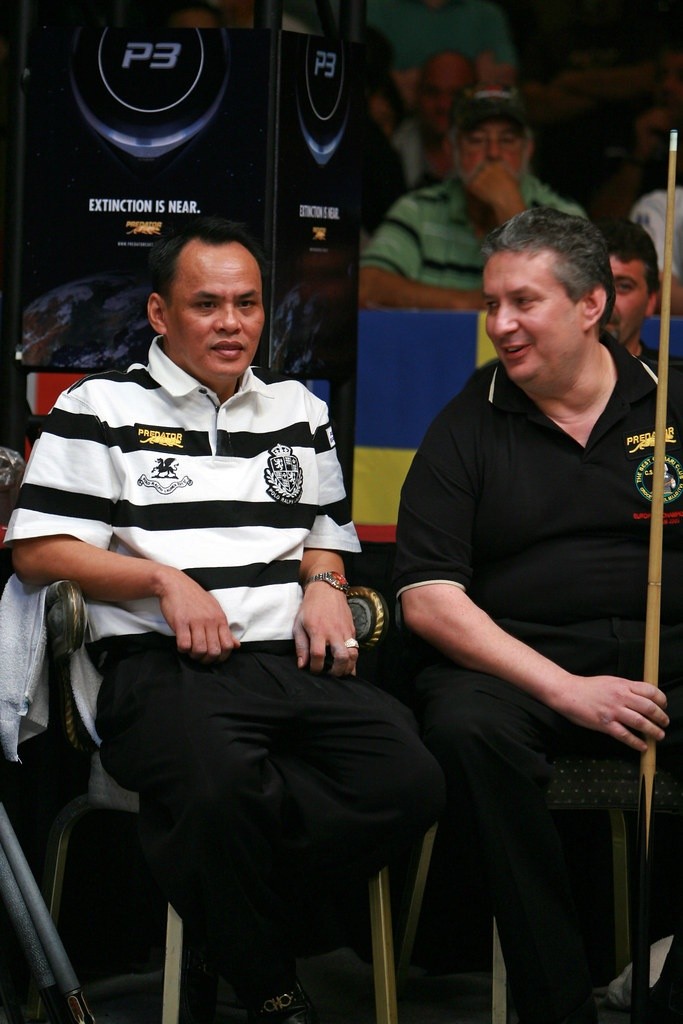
top-left (0, 446), bottom-right (397, 1024)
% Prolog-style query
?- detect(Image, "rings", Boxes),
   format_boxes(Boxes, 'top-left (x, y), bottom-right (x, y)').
top-left (344, 639), bottom-right (359, 649)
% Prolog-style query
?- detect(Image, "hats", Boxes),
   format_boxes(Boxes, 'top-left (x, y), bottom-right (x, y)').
top-left (446, 81), bottom-right (529, 132)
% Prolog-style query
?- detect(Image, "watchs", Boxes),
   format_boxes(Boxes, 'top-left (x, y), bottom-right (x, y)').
top-left (303, 570), bottom-right (350, 593)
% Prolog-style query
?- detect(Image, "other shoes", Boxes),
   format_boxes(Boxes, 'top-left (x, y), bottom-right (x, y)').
top-left (180, 926), bottom-right (218, 1024)
top-left (244, 977), bottom-right (318, 1024)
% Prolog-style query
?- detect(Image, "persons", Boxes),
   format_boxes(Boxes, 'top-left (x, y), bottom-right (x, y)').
top-left (168, 0), bottom-right (682, 372)
top-left (396, 209), bottom-right (682, 1024)
top-left (2, 224), bottom-right (445, 1024)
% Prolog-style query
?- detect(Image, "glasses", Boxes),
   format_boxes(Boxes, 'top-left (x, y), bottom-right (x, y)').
top-left (447, 125), bottom-right (529, 154)
